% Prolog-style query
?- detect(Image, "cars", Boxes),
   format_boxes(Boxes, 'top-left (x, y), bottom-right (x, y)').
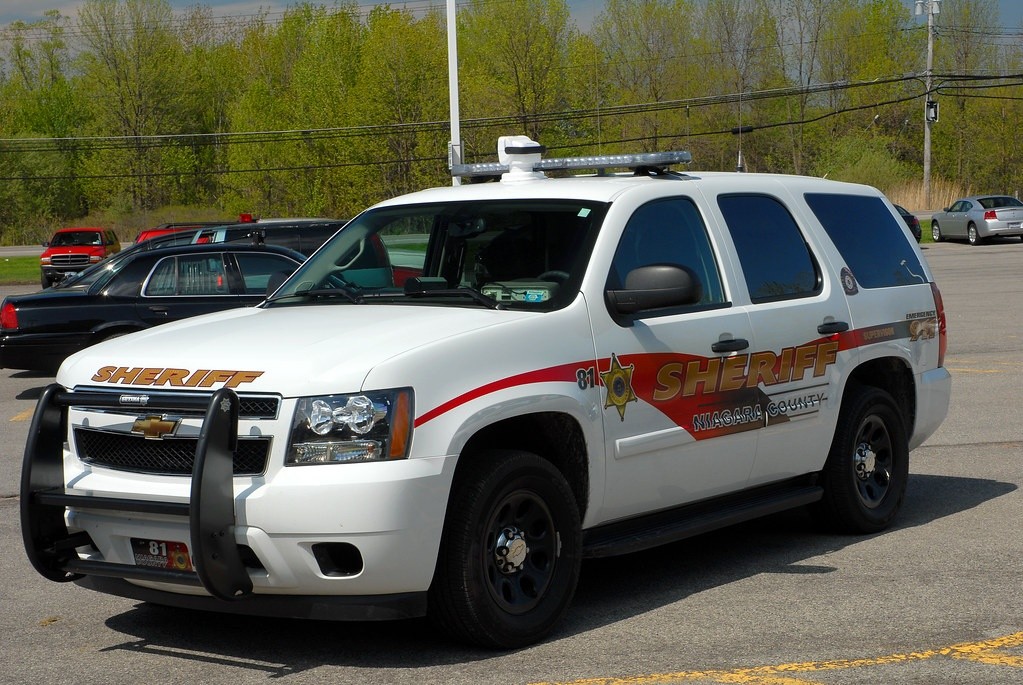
top-left (0, 238), bottom-right (361, 372)
top-left (893, 203), bottom-right (923, 244)
top-left (931, 195), bottom-right (1023, 246)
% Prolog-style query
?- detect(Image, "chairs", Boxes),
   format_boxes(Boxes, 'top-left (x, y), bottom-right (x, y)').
top-left (604, 211), bottom-right (692, 302)
top-left (477, 227), bottom-right (554, 294)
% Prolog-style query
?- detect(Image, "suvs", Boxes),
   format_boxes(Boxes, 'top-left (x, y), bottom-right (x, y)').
top-left (40, 227), bottom-right (122, 289)
top-left (17, 132), bottom-right (954, 660)
top-left (129, 214), bottom-right (431, 293)
